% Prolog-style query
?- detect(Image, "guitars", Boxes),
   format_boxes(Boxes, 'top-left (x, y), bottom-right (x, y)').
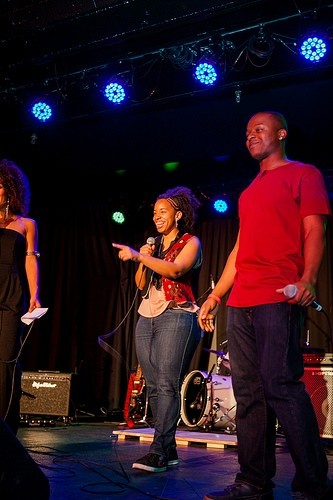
top-left (123, 364), bottom-right (153, 429)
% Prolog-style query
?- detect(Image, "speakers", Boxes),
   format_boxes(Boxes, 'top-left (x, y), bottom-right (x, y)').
top-left (301, 367), bottom-right (333, 439)
top-left (18, 371), bottom-right (72, 418)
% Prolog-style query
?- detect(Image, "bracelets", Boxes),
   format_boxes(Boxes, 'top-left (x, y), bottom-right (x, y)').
top-left (207, 294), bottom-right (221, 308)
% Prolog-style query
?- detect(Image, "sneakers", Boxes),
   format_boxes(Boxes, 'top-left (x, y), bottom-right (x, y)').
top-left (204, 481), bottom-right (274, 500)
top-left (165, 450), bottom-right (179, 465)
top-left (294, 476), bottom-right (333, 500)
top-left (132, 453), bottom-right (167, 472)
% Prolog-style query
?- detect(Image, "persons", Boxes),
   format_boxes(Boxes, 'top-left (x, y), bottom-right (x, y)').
top-left (0, 158), bottom-right (51, 500)
top-left (198, 106), bottom-right (333, 500)
top-left (112, 187), bottom-right (205, 472)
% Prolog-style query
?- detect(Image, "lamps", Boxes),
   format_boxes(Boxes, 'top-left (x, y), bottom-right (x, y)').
top-left (192, 36), bottom-right (233, 91)
top-left (98, 64), bottom-right (134, 105)
top-left (26, 86), bottom-right (67, 122)
top-left (247, 23), bottom-right (276, 66)
top-left (112, 205), bottom-right (130, 224)
top-left (211, 187), bottom-right (231, 215)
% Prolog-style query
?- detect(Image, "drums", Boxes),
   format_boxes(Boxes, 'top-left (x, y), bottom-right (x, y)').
top-left (180, 369), bottom-right (237, 434)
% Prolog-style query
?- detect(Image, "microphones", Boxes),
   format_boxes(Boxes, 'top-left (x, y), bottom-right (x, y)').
top-left (211, 275), bottom-right (215, 291)
top-left (142, 237), bottom-right (155, 272)
top-left (284, 284), bottom-right (322, 312)
